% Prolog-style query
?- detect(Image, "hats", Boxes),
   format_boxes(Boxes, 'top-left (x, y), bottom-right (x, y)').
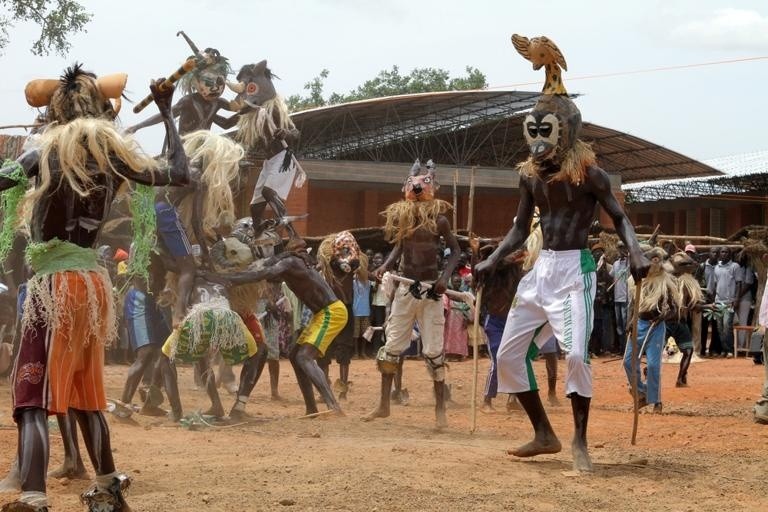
top-left (659, 238), bottom-right (678, 252)
top-left (592, 244), bottom-right (605, 253)
top-left (685, 245), bottom-right (696, 254)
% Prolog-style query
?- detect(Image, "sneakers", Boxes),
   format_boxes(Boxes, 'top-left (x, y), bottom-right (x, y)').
top-left (727, 353), bottom-right (733, 358)
top-left (721, 351), bottom-right (725, 356)
top-left (711, 353), bottom-right (719, 356)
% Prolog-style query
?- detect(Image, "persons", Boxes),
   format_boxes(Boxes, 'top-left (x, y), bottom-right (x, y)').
top-left (198, 237), bottom-right (348, 423)
top-left (729, 248), bottom-right (753, 358)
top-left (443, 272), bottom-right (471, 363)
top-left (108, 233), bottom-right (187, 425)
top-left (370, 252), bottom-right (390, 356)
top-left (317, 232), bottom-right (364, 403)
top-left (121, 30), bottom-right (246, 237)
top-left (263, 277), bottom-right (288, 399)
top-left (684, 242), bottom-right (704, 357)
top-left (589, 241), bottom-right (618, 359)
top-left (0, 259), bottom-right (93, 497)
top-left (362, 158), bottom-right (462, 433)
top-left (745, 223), bottom-right (768, 425)
top-left (364, 248), bottom-right (374, 272)
top-left (0, 64), bottom-right (191, 512)
top-left (609, 239), bottom-right (631, 354)
top-left (620, 224), bottom-right (682, 412)
top-left (508, 328), bottom-right (558, 406)
top-left (481, 243), bottom-right (522, 411)
top-left (181, 231), bottom-right (268, 423)
top-left (701, 244), bottom-right (720, 357)
top-left (714, 246), bottom-right (742, 359)
top-left (211, 60), bottom-right (302, 240)
top-left (458, 250), bottom-right (470, 279)
top-left (354, 263), bottom-right (374, 362)
top-left (470, 35), bottom-right (654, 475)
